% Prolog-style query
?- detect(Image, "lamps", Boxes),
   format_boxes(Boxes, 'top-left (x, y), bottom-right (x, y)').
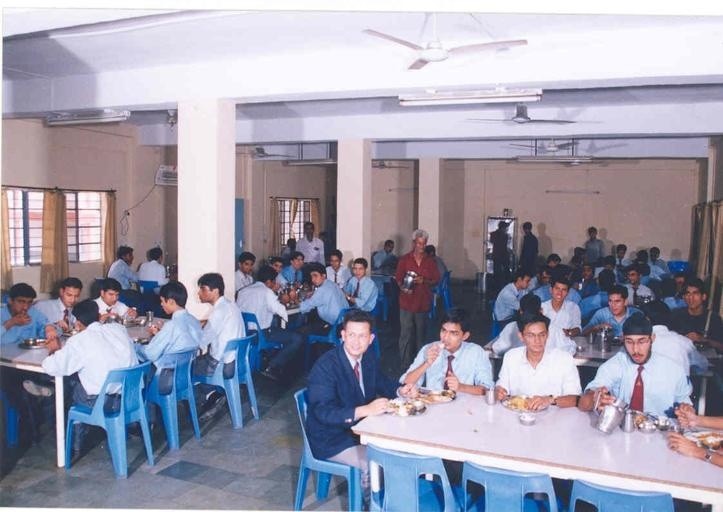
top-left (395, 87), bottom-right (544, 108)
top-left (513, 138), bottom-right (594, 165)
top-left (45, 109), bottom-right (130, 127)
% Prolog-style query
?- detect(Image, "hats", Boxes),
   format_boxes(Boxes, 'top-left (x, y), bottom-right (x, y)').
top-left (622, 311), bottom-right (653, 336)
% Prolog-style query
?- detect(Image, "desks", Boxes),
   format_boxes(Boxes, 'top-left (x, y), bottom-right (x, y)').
top-left (1, 315), bottom-right (171, 469)
top-left (482, 335), bottom-right (722, 417)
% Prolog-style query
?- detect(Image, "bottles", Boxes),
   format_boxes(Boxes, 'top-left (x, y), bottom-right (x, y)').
top-left (165, 264), bottom-right (177, 277)
top-left (593, 392), bottom-right (685, 434)
top-left (403, 271), bottom-right (417, 290)
top-left (107, 308), bottom-right (153, 344)
top-left (282, 281), bottom-right (304, 307)
top-left (518, 412), bottom-right (536, 426)
top-left (590, 327), bottom-right (614, 352)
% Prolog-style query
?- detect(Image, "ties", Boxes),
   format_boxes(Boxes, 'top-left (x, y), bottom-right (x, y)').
top-left (628, 365), bottom-right (645, 413)
top-left (353, 362), bottom-right (360, 382)
top-left (63, 309), bottom-right (70, 327)
top-left (334, 273), bottom-right (337, 283)
top-left (443, 355), bottom-right (455, 390)
top-left (106, 308), bottom-right (112, 313)
top-left (632, 288), bottom-right (640, 305)
top-left (353, 282), bottom-right (360, 298)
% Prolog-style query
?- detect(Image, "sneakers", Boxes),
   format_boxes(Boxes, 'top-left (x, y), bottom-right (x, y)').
top-left (21, 380), bottom-right (56, 398)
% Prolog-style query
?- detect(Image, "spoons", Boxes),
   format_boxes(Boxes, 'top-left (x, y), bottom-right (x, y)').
top-left (673, 405), bottom-right (697, 432)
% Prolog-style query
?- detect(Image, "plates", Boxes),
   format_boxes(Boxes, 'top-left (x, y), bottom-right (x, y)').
top-left (18, 338), bottom-right (51, 348)
top-left (501, 396), bottom-right (549, 413)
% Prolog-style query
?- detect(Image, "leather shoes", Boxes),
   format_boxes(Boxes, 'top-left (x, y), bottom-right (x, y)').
top-left (198, 392), bottom-right (226, 424)
top-left (262, 366), bottom-right (286, 381)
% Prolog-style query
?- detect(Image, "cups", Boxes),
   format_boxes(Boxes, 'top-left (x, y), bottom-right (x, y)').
top-left (576, 345), bottom-right (585, 352)
top-left (383, 387), bottom-right (456, 416)
top-left (486, 384), bottom-right (496, 405)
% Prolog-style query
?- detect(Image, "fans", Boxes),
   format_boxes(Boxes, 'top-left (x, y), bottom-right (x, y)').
top-left (463, 105), bottom-right (578, 125)
top-left (234, 145), bottom-right (297, 160)
top-left (360, 18), bottom-right (527, 70)
top-left (508, 137), bottom-right (580, 153)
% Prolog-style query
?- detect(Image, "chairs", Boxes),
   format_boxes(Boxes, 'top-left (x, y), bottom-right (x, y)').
top-left (668, 261), bottom-right (691, 272)
top-left (118, 280), bottom-right (165, 318)
top-left (89, 279), bottom-right (103, 299)
top-left (63, 333), bottom-right (261, 481)
top-left (486, 299), bottom-right (502, 338)
top-left (372, 274), bottom-right (387, 321)
top-left (240, 303), bottom-right (380, 374)
top-left (2, 392), bottom-right (20, 448)
top-left (295, 385), bottom-right (723, 512)
top-left (429, 271), bottom-right (452, 320)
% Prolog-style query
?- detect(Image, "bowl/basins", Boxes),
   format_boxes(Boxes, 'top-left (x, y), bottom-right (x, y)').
top-left (699, 432), bottom-right (722, 449)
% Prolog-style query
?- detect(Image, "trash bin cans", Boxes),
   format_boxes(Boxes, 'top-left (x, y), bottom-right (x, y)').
top-left (474, 272), bottom-right (492, 295)
